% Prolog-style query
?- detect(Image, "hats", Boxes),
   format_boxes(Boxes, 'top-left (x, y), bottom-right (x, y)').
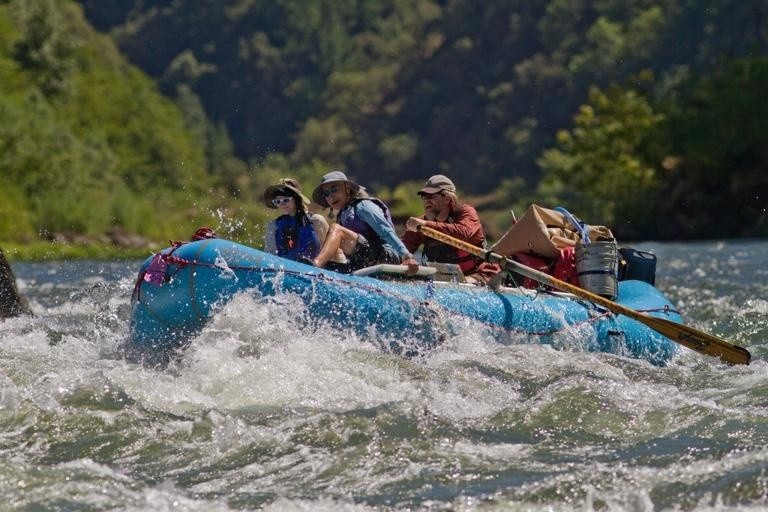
top-left (264, 177), bottom-right (311, 209)
top-left (312, 171), bottom-right (360, 208)
top-left (417, 174), bottom-right (455, 195)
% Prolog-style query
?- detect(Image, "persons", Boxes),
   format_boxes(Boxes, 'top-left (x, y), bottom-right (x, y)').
top-left (400, 174), bottom-right (487, 277)
top-left (263, 178), bottom-right (347, 271)
top-left (302, 170), bottom-right (420, 275)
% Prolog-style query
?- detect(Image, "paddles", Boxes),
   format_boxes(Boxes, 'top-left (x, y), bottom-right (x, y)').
top-left (415, 225), bottom-right (751, 367)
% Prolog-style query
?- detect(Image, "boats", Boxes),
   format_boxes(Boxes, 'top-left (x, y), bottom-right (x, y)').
top-left (127, 236), bottom-right (687, 376)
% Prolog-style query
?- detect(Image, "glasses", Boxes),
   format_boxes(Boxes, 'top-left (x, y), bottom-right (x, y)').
top-left (323, 186), bottom-right (338, 196)
top-left (271, 197), bottom-right (292, 205)
